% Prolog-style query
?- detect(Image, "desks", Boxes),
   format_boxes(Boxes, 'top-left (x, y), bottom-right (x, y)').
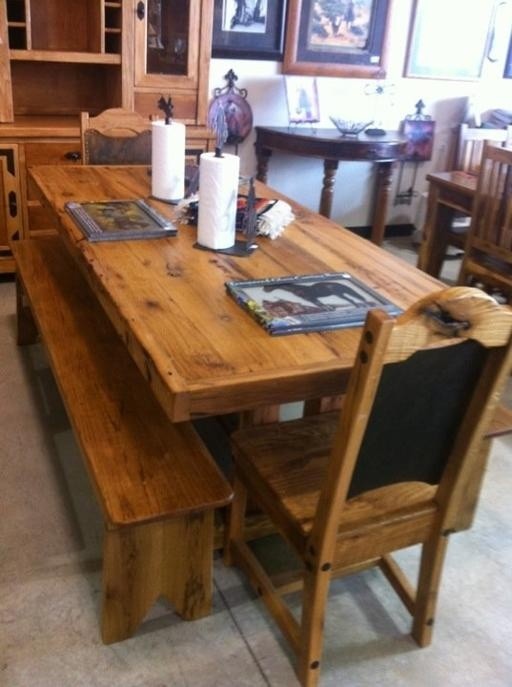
top-left (252, 118), bottom-right (417, 250)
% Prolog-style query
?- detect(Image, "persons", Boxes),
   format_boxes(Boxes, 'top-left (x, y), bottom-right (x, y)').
top-left (332, 11), bottom-right (342, 36)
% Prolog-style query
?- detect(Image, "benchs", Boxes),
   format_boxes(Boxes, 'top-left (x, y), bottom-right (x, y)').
top-left (5, 236), bottom-right (241, 649)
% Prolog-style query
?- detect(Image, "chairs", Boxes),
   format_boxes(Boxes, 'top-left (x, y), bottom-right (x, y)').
top-left (79, 108), bottom-right (191, 169)
top-left (451, 138), bottom-right (512, 301)
top-left (226, 284), bottom-right (512, 686)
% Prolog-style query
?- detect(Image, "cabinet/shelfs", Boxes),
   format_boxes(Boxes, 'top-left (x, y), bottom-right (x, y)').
top-left (1, 143), bottom-right (218, 275)
top-left (130, 1), bottom-right (202, 129)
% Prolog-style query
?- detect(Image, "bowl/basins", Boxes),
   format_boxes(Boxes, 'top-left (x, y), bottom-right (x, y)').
top-left (329, 112), bottom-right (375, 136)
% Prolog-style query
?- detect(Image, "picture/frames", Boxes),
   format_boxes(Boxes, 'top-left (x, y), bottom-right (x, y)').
top-left (280, 1), bottom-right (393, 78)
top-left (284, 76), bottom-right (319, 123)
top-left (62, 195), bottom-right (178, 243)
top-left (210, 0), bottom-right (284, 65)
top-left (401, 0), bottom-right (489, 80)
top-left (223, 271), bottom-right (406, 337)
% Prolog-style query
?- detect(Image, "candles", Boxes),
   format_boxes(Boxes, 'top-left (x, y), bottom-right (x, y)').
top-left (150, 117), bottom-right (186, 202)
top-left (196, 152), bottom-right (243, 251)
